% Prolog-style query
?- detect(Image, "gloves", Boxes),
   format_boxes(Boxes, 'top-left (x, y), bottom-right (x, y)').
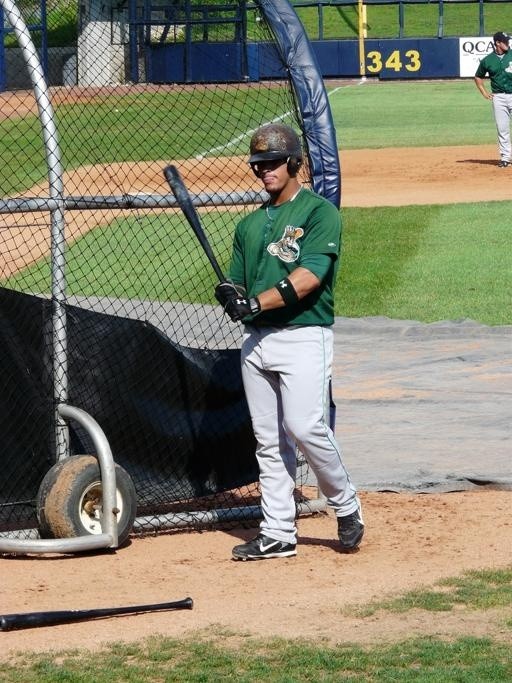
top-left (214, 279), bottom-right (262, 323)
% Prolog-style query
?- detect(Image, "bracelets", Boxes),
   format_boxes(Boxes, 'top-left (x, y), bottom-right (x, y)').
top-left (273, 276), bottom-right (299, 307)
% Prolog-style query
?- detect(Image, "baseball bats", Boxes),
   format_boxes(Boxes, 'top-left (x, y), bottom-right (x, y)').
top-left (2, 597), bottom-right (194, 633)
top-left (162, 165), bottom-right (254, 325)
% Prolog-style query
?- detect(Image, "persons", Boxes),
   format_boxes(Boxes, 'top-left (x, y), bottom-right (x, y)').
top-left (213, 122), bottom-right (367, 562)
top-left (474, 31), bottom-right (512, 167)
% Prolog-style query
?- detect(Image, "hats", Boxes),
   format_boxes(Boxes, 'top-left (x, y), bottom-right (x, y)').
top-left (494, 31), bottom-right (512, 42)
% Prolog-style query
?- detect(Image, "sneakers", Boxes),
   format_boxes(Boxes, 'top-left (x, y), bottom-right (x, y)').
top-left (496, 161), bottom-right (511, 168)
top-left (336, 496), bottom-right (365, 550)
top-left (231, 532), bottom-right (296, 561)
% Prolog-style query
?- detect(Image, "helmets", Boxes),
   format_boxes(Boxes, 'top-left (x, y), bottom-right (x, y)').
top-left (245, 122), bottom-right (303, 173)
